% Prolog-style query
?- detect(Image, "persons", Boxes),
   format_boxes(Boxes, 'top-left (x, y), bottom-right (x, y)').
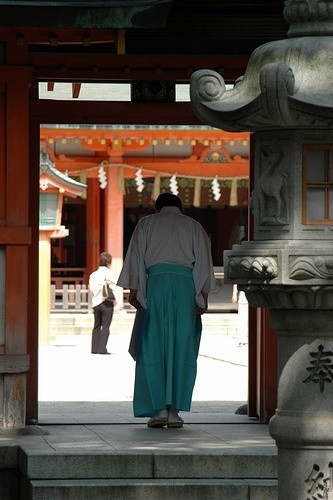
top-left (117, 192), bottom-right (218, 428)
top-left (88, 253), bottom-right (117, 354)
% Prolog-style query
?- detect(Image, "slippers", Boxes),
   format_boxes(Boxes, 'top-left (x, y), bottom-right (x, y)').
top-left (147, 415), bottom-right (167, 427)
top-left (166, 417), bottom-right (184, 428)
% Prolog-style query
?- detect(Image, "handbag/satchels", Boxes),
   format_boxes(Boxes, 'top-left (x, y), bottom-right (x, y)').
top-left (101, 275), bottom-right (116, 301)
